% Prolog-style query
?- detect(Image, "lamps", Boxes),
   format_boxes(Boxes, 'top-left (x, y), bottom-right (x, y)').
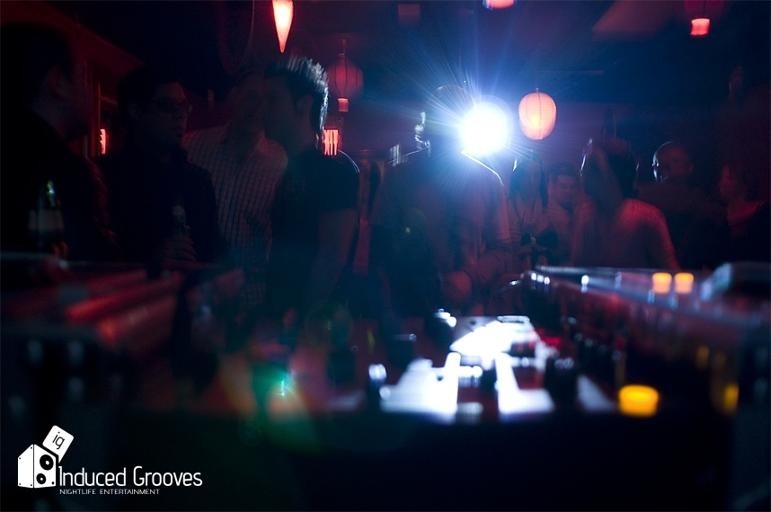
top-left (325, 38), bottom-right (363, 112)
top-left (517, 88), bottom-right (558, 141)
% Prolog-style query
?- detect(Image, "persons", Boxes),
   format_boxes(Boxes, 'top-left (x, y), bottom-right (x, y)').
top-left (45, 56), bottom-right (770, 349)
